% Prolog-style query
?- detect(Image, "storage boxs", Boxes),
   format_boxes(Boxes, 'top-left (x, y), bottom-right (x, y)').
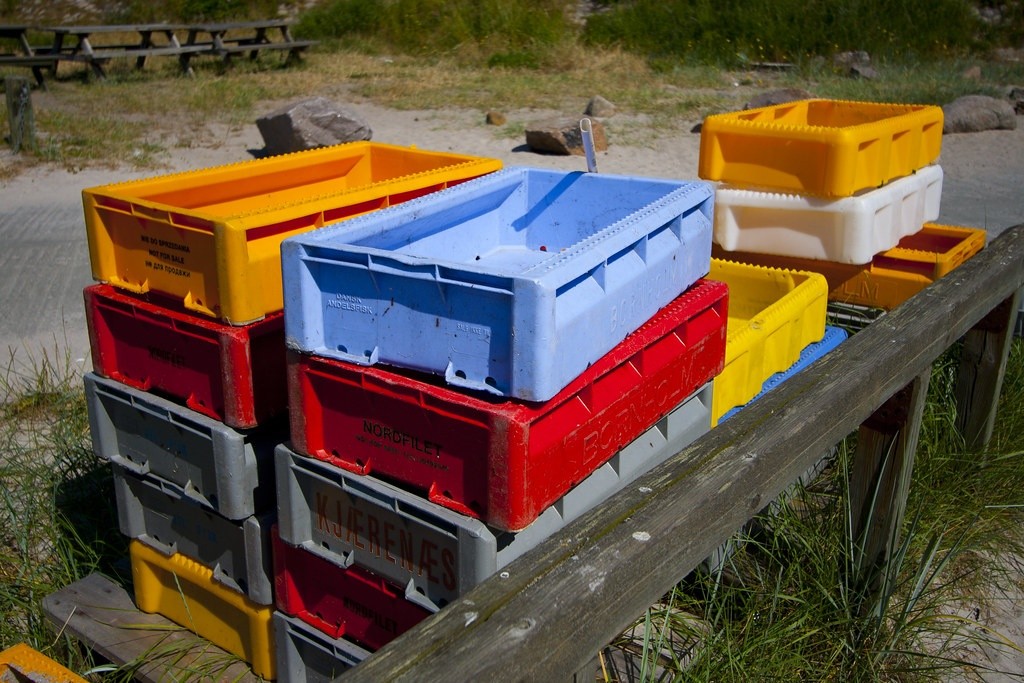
top-left (697, 98), bottom-right (943, 199)
top-left (81, 139), bottom-right (850, 683)
top-left (712, 222), bottom-right (987, 309)
top-left (714, 164), bottom-right (943, 265)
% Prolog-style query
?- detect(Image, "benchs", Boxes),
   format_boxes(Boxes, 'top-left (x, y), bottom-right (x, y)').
top-left (0, 37), bottom-right (321, 92)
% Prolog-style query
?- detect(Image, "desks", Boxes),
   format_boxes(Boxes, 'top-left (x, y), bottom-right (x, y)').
top-left (35, 24), bottom-right (196, 83)
top-left (0, 24), bottom-right (50, 92)
top-left (180, 18), bottom-right (304, 77)
